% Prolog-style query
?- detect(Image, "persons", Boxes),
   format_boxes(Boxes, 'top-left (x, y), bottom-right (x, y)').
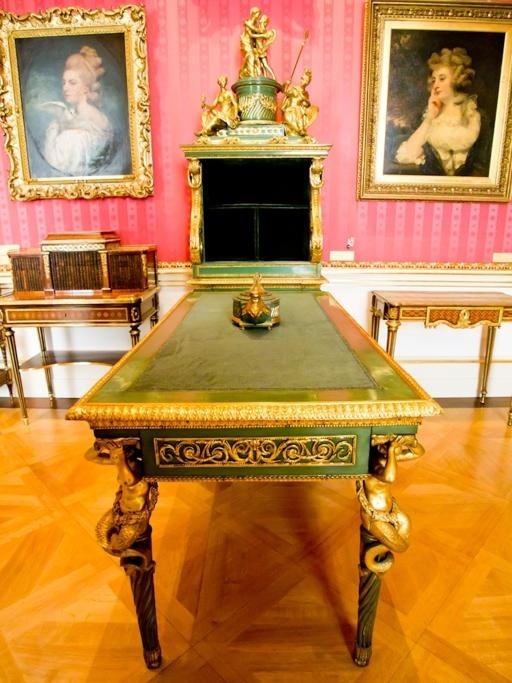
top-left (284, 68), bottom-right (319, 137)
top-left (193, 76), bottom-right (239, 137)
top-left (236, 7), bottom-right (272, 77)
top-left (393, 46), bottom-right (488, 175)
top-left (243, 16), bottom-right (277, 79)
top-left (42, 45), bottom-right (115, 177)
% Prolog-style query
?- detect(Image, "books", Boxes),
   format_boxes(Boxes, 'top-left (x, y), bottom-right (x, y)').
top-left (40, 230), bottom-right (121, 252)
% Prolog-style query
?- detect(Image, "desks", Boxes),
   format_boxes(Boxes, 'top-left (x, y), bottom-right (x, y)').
top-left (372, 290), bottom-right (512, 426)
top-left (70, 288), bottom-right (437, 672)
top-left (2, 287), bottom-right (159, 425)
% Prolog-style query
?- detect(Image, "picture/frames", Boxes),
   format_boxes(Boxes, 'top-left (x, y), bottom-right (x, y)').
top-left (356, 1), bottom-right (512, 206)
top-left (1, 2), bottom-right (154, 203)
